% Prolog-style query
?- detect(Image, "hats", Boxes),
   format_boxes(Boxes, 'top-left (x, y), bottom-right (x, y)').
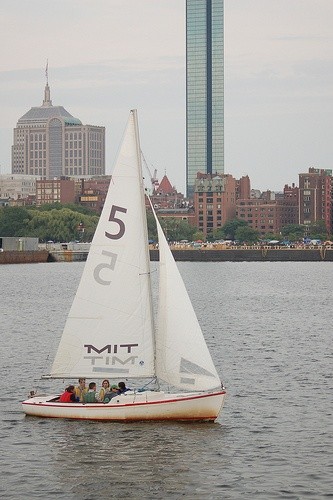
top-left (111, 385), bottom-right (120, 389)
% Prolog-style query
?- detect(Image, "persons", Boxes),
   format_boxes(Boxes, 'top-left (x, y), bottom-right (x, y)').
top-left (74, 377), bottom-right (130, 404)
top-left (60, 385), bottom-right (80, 403)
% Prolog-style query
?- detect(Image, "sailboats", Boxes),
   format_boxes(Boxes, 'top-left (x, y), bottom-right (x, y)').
top-left (20, 106), bottom-right (228, 424)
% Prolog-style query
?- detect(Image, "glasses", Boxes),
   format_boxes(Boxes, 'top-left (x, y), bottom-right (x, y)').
top-left (116, 389), bottom-right (118, 390)
